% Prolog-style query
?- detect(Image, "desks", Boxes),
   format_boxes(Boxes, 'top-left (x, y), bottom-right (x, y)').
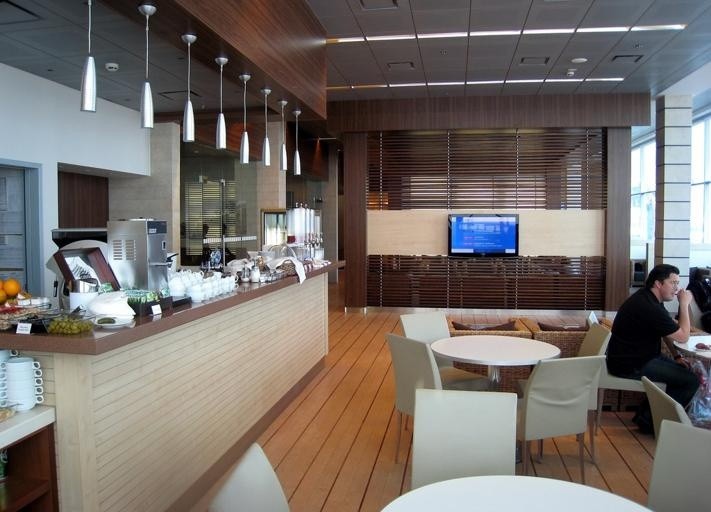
top-left (667, 332), bottom-right (709, 424)
top-left (372, 473), bottom-right (663, 512)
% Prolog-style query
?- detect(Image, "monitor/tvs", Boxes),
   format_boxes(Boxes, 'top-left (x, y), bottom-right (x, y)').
top-left (448, 213), bottom-right (519, 256)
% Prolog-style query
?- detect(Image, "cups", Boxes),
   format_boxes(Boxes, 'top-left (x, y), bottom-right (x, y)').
top-left (169, 268), bottom-right (240, 303)
top-left (0, 348), bottom-right (45, 413)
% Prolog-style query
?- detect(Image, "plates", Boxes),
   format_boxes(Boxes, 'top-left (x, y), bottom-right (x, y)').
top-left (92, 315), bottom-right (132, 329)
top-left (695, 344), bottom-right (711, 351)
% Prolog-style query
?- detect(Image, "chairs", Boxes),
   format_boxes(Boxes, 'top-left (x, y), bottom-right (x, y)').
top-left (412, 386), bottom-right (520, 494)
top-left (586, 310), bottom-right (669, 438)
top-left (640, 374), bottom-right (694, 441)
top-left (643, 416), bottom-right (711, 512)
top-left (440, 313), bottom-right (710, 413)
top-left (203, 440), bottom-right (293, 511)
top-left (400, 311), bottom-right (492, 392)
top-left (386, 330), bottom-right (497, 471)
top-left (517, 323), bottom-right (612, 467)
top-left (515, 355), bottom-right (606, 487)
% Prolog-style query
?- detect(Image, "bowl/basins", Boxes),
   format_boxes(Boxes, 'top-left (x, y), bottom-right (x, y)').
top-left (42, 314), bottom-right (97, 336)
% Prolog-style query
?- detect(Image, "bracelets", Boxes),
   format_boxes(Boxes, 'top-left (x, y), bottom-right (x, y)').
top-left (673, 354), bottom-right (681, 359)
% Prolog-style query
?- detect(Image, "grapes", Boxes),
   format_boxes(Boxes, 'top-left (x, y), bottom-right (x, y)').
top-left (47, 317), bottom-right (91, 335)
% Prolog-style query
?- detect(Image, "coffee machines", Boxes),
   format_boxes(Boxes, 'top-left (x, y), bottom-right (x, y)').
top-left (107, 216), bottom-right (180, 297)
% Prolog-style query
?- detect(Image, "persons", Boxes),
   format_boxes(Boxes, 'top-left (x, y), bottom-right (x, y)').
top-left (603, 264), bottom-right (700, 436)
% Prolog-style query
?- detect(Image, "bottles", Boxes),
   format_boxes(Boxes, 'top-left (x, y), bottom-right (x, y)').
top-left (241, 266), bottom-right (251, 282)
top-left (251, 265), bottom-right (261, 283)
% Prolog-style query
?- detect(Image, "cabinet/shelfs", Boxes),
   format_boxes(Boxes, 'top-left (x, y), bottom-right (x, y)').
top-left (0, 403), bottom-right (65, 511)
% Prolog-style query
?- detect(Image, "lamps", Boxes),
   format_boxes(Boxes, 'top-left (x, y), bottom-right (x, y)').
top-left (181, 29), bottom-right (198, 144)
top-left (138, 5), bottom-right (158, 131)
top-left (214, 54), bottom-right (305, 179)
top-left (77, 3), bottom-right (101, 116)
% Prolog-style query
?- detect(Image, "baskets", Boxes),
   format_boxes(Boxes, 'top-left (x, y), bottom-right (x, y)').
top-left (280, 260), bottom-right (296, 276)
